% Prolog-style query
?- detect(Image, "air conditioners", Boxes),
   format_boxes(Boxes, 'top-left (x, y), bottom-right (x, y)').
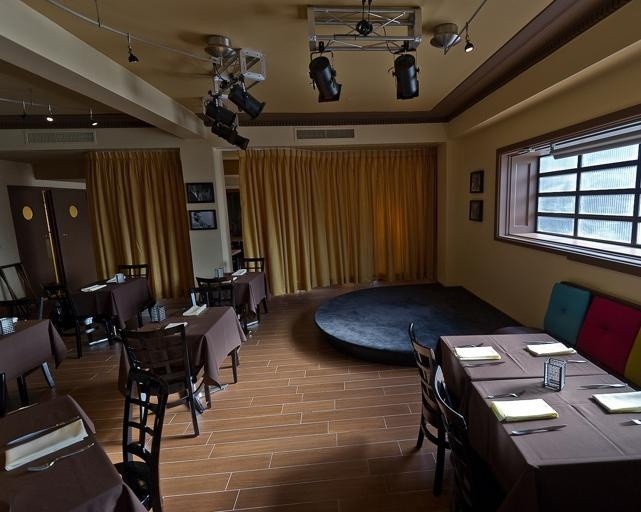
top-left (25, 130), bottom-right (97, 147)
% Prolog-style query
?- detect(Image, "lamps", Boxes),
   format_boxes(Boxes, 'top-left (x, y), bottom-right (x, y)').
top-left (464, 22), bottom-right (474, 54)
top-left (195, 32), bottom-right (270, 153)
top-left (307, 0), bottom-right (423, 103)
top-left (125, 31), bottom-right (141, 65)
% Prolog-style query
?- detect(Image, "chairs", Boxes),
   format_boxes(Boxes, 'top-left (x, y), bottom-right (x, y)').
top-left (625, 326), bottom-right (641, 386)
top-left (237, 256), bottom-right (269, 323)
top-left (408, 321), bottom-right (449, 469)
top-left (189, 285), bottom-right (239, 383)
top-left (493, 283), bottom-right (591, 341)
top-left (197, 275), bottom-right (241, 382)
top-left (577, 294), bottom-right (641, 377)
top-left (46, 284), bottom-right (113, 357)
top-left (113, 263), bottom-right (154, 329)
top-left (1, 262), bottom-right (44, 316)
top-left (123, 322), bottom-right (199, 437)
top-left (110, 367), bottom-right (170, 512)
top-left (433, 364), bottom-right (500, 512)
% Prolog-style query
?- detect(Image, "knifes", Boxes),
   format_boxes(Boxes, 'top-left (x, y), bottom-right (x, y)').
top-left (7, 415), bottom-right (80, 446)
top-left (463, 361), bottom-right (505, 368)
top-left (580, 384), bottom-right (628, 388)
top-left (512, 425), bottom-right (567, 435)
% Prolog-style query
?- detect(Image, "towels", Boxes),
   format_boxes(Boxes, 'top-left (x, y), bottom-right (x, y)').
top-left (3, 419), bottom-right (88, 472)
top-left (452, 346), bottom-right (501, 362)
top-left (182, 303), bottom-right (207, 317)
top-left (221, 276), bottom-right (239, 286)
top-left (81, 283), bottom-right (108, 293)
top-left (163, 321), bottom-right (189, 336)
top-left (592, 391), bottom-right (641, 414)
top-left (489, 397), bottom-right (560, 422)
top-left (106, 275), bottom-right (127, 284)
top-left (524, 341), bottom-right (578, 357)
top-left (231, 268), bottom-right (248, 276)
top-left (0, 317), bottom-right (19, 324)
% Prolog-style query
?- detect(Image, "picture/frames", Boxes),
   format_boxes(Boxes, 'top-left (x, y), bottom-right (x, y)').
top-left (185, 180), bottom-right (215, 205)
top-left (189, 209), bottom-right (216, 231)
top-left (470, 169), bottom-right (483, 193)
top-left (469, 199), bottom-right (483, 221)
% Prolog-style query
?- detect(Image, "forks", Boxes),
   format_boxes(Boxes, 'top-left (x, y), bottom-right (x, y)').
top-left (487, 390), bottom-right (526, 399)
top-left (454, 342), bottom-right (484, 348)
top-left (27, 442), bottom-right (95, 471)
top-left (628, 416), bottom-right (641, 425)
